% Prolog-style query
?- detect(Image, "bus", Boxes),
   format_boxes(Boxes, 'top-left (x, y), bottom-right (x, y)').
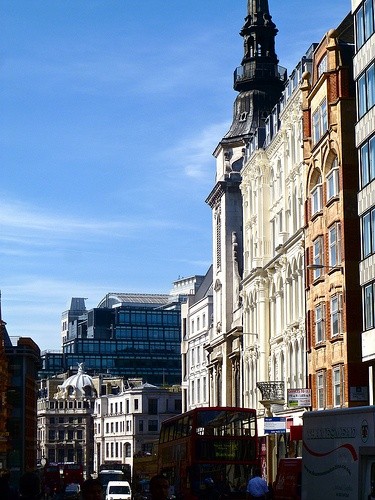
top-left (158, 407), bottom-right (260, 500)
top-left (44, 462), bottom-right (83, 500)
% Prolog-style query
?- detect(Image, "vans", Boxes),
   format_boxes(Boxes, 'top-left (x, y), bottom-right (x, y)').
top-left (104, 480), bottom-right (132, 500)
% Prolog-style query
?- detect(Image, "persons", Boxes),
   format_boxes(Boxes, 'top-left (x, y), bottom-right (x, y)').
top-left (149, 474), bottom-right (170, 500)
top-left (245, 468), bottom-right (270, 500)
top-left (285, 446), bottom-right (298, 458)
top-left (83, 480), bottom-right (104, 500)
top-left (18, 473), bottom-right (43, 500)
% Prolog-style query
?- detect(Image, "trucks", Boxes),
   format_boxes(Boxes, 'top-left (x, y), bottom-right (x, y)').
top-left (272, 404), bottom-right (375, 500)
